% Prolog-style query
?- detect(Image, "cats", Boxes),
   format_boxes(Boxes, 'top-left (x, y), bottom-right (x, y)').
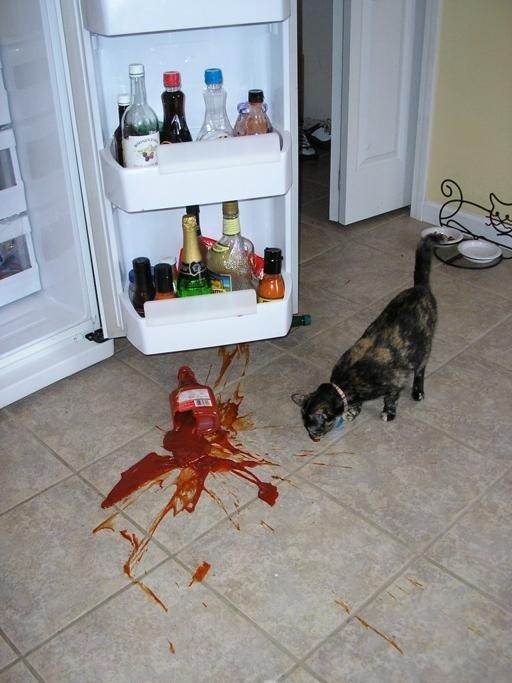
top-left (291, 231), bottom-right (445, 440)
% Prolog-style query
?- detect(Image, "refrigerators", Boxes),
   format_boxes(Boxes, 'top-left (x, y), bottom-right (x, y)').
top-left (0, 1), bottom-right (302, 406)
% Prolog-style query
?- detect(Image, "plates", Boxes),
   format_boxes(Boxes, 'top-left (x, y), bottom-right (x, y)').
top-left (456, 239), bottom-right (501, 265)
top-left (420, 224), bottom-right (462, 248)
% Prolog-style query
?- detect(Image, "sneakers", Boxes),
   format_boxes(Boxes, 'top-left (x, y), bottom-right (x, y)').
top-left (298, 121), bottom-right (331, 159)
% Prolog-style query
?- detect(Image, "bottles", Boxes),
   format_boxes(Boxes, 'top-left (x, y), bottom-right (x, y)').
top-left (172, 366), bottom-right (221, 443)
top-left (290, 314), bottom-right (311, 327)
top-left (129, 203), bottom-right (284, 323)
top-left (109, 63), bottom-right (270, 167)
